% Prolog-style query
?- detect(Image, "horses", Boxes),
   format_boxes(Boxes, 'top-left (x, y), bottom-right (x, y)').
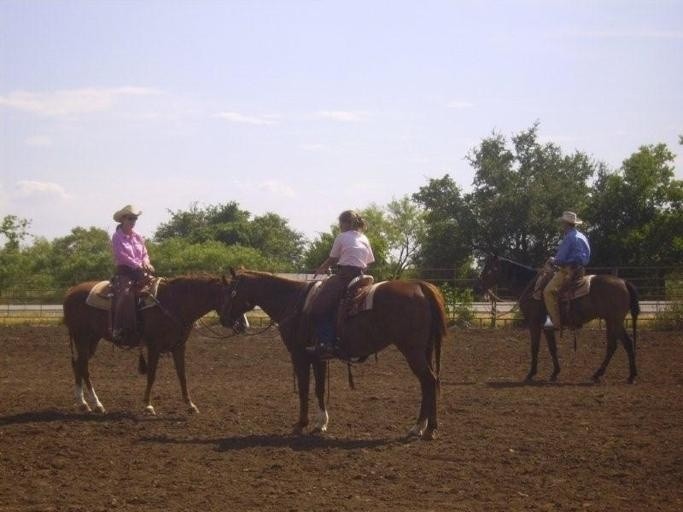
top-left (472, 249), bottom-right (640, 385)
top-left (218, 263), bottom-right (448, 441)
top-left (62, 273), bottom-right (250, 417)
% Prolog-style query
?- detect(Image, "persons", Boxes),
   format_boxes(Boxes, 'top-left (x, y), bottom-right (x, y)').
top-left (304, 209), bottom-right (375, 356)
top-left (109, 205), bottom-right (156, 347)
top-left (542, 210), bottom-right (592, 332)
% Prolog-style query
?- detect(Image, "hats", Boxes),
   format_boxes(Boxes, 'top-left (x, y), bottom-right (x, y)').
top-left (553, 210), bottom-right (583, 224)
top-left (113, 205), bottom-right (143, 222)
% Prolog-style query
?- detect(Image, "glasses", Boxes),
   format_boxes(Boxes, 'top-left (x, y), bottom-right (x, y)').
top-left (125, 216), bottom-right (138, 221)
